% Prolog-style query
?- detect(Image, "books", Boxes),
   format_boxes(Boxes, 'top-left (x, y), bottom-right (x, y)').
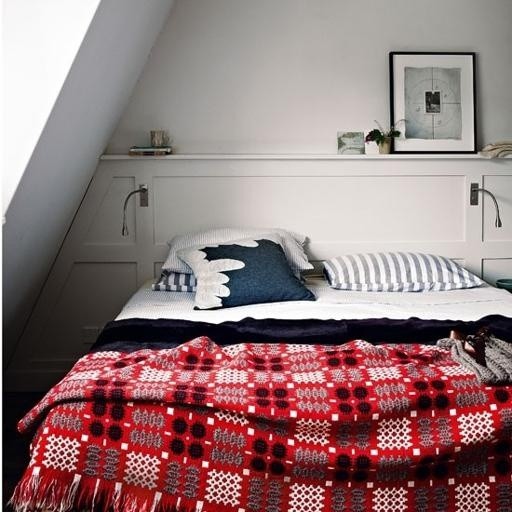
top-left (130, 145), bottom-right (172, 154)
top-left (128, 150), bottom-right (172, 156)
top-left (476, 139), bottom-right (512, 160)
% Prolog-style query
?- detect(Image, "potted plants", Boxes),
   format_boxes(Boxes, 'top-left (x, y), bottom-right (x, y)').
top-left (366, 117), bottom-right (407, 155)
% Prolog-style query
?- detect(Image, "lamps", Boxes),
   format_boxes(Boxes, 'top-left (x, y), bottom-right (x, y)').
top-left (469, 183), bottom-right (502, 228)
top-left (121, 184), bottom-right (148, 237)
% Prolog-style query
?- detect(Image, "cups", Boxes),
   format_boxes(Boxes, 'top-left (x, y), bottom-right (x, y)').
top-left (151, 129), bottom-right (169, 147)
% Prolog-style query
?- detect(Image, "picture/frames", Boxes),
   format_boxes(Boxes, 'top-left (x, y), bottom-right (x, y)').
top-left (389, 51), bottom-right (478, 154)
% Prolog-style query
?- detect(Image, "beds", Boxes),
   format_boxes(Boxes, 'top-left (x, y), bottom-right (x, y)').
top-left (24, 275), bottom-right (511, 511)
